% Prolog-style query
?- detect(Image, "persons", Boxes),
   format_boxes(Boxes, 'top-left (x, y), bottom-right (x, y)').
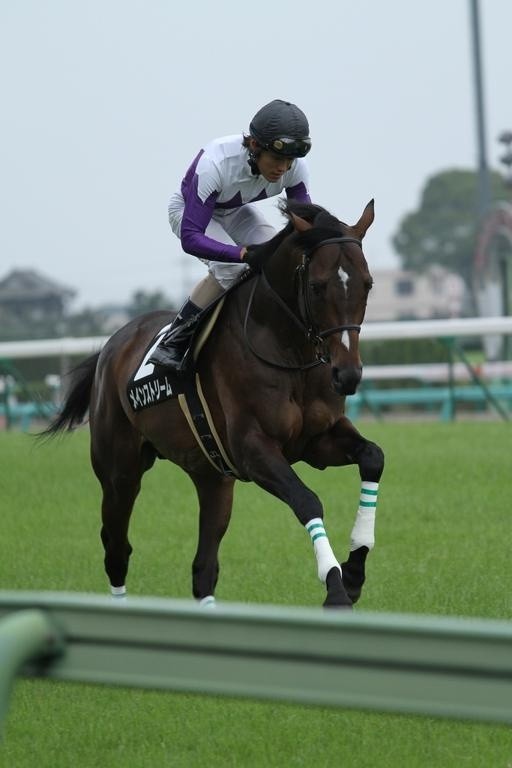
top-left (144, 99), bottom-right (313, 377)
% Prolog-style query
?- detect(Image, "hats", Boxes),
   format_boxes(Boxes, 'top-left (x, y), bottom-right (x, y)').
top-left (249, 100), bottom-right (311, 158)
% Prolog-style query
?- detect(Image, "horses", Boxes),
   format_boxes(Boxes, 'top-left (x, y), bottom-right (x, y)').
top-left (22, 197), bottom-right (385, 610)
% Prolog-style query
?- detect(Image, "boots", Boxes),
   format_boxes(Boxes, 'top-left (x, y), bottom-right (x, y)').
top-left (147, 295), bottom-right (209, 372)
